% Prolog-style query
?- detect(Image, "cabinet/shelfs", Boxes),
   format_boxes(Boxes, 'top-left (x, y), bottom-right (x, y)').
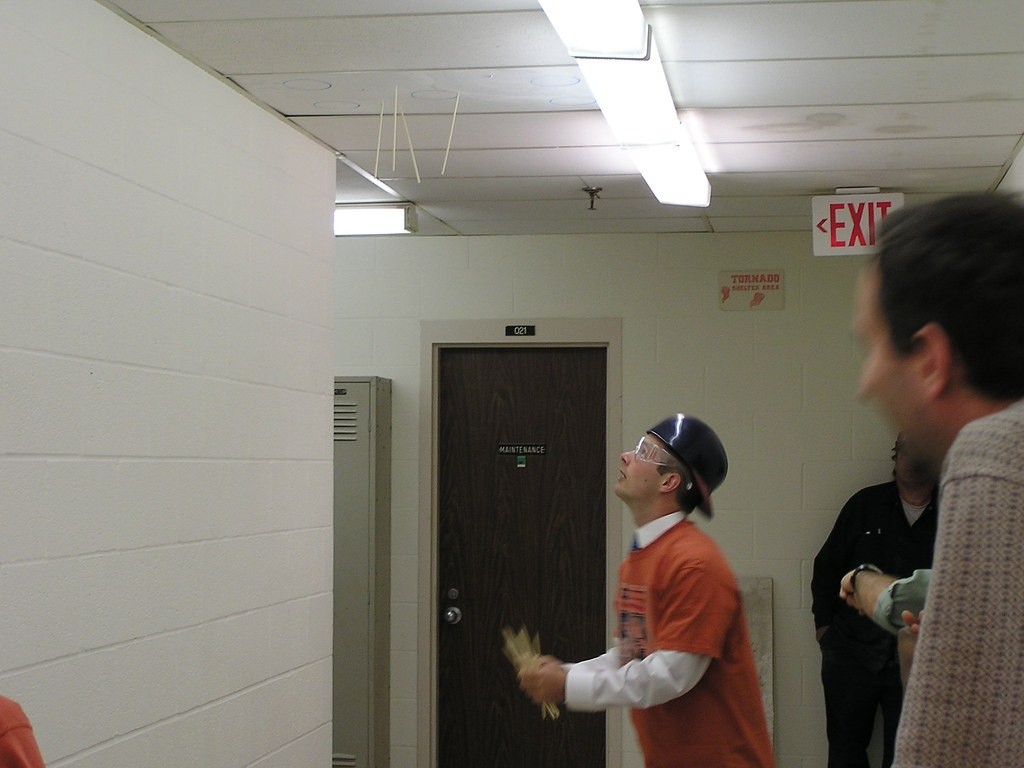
top-left (331, 374), bottom-right (392, 768)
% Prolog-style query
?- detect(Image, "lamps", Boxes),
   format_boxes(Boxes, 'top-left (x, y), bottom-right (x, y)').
top-left (538, 0), bottom-right (712, 209)
top-left (334, 202), bottom-right (419, 237)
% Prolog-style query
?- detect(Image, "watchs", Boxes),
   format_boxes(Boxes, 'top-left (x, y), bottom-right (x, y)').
top-left (849, 563), bottom-right (884, 588)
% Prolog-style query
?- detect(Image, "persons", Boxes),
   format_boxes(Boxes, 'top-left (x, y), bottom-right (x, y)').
top-left (838, 562), bottom-right (933, 636)
top-left (517, 414), bottom-right (778, 767)
top-left (808, 432), bottom-right (942, 767)
top-left (851, 187), bottom-right (1022, 768)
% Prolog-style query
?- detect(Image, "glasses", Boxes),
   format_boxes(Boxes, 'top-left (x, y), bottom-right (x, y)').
top-left (634, 437), bottom-right (692, 490)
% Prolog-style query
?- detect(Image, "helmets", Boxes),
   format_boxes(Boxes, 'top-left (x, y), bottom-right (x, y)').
top-left (647, 413), bottom-right (728, 518)
